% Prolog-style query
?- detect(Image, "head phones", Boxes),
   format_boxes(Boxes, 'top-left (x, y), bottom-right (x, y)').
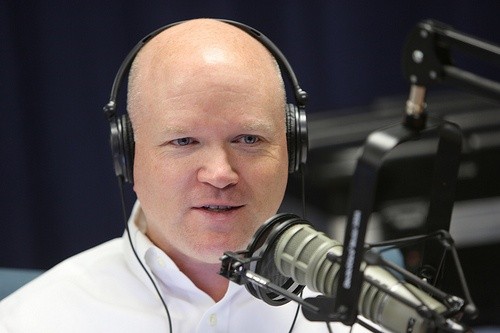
top-left (103, 18), bottom-right (310, 196)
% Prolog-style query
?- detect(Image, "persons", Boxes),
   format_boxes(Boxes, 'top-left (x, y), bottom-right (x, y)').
top-left (0, 16), bottom-right (355, 333)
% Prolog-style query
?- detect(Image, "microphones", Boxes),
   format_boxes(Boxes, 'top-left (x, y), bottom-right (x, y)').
top-left (272, 219), bottom-right (466, 333)
